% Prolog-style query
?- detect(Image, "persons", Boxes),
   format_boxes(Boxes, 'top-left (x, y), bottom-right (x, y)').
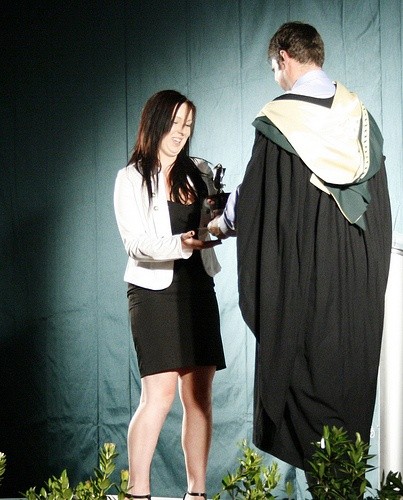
top-left (113, 90), bottom-right (226, 500)
top-left (206, 21), bottom-right (392, 500)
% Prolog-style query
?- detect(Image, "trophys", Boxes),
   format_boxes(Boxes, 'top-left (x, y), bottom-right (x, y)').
top-left (198, 163), bottom-right (231, 248)
